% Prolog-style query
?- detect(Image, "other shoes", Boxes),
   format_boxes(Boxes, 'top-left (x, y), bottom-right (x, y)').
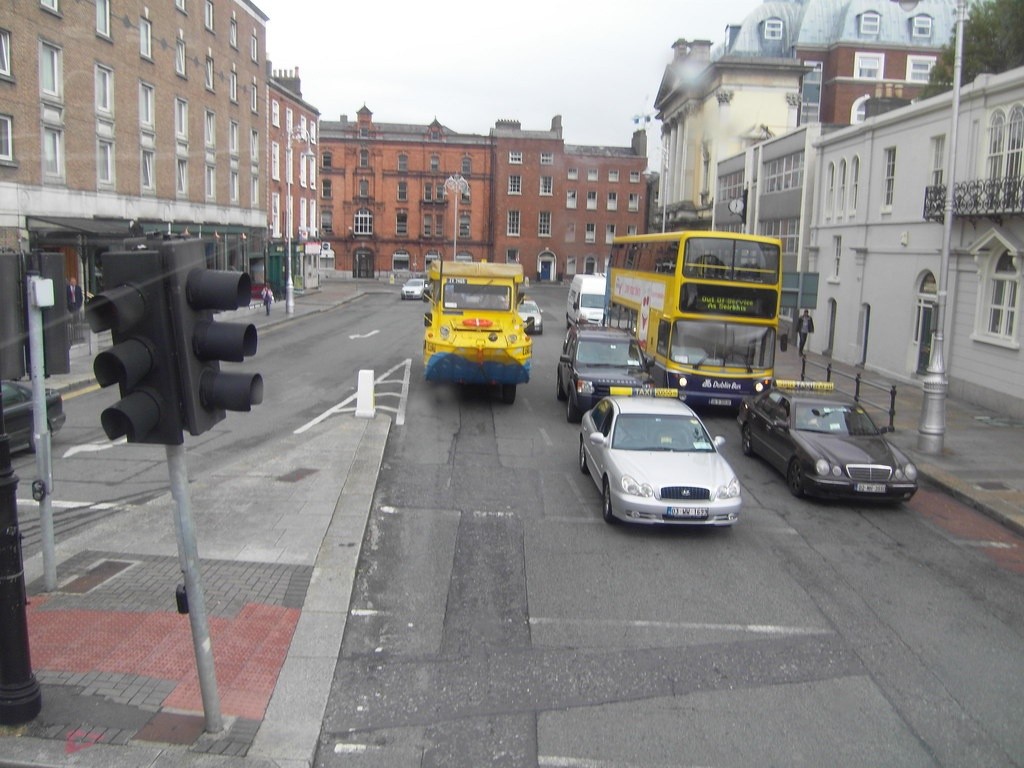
top-left (799, 352), bottom-right (803, 356)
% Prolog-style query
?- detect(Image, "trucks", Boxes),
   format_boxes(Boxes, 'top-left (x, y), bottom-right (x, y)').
top-left (422, 258), bottom-right (537, 405)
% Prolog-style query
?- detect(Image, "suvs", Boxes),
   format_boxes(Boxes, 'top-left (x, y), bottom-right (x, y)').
top-left (557, 324), bottom-right (656, 424)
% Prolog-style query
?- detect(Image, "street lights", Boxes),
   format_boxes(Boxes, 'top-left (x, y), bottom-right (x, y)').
top-left (444, 175), bottom-right (469, 262)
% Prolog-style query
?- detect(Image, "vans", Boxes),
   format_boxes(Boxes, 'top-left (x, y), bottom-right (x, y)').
top-left (566, 273), bottom-right (608, 330)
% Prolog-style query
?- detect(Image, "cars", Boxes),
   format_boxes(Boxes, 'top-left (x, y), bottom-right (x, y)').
top-left (0, 379), bottom-right (66, 456)
top-left (735, 378), bottom-right (919, 507)
top-left (579, 386), bottom-right (742, 527)
top-left (518, 300), bottom-right (544, 335)
top-left (401, 278), bottom-right (428, 300)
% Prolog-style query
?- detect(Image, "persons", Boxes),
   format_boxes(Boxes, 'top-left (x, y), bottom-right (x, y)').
top-left (261, 282), bottom-right (274, 317)
top-left (67, 279), bottom-right (82, 323)
top-left (786, 409), bottom-right (817, 427)
top-left (797, 310), bottom-right (815, 360)
top-left (604, 419), bottom-right (636, 446)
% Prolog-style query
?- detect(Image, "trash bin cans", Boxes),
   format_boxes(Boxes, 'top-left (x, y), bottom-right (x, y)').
top-left (558, 273), bottom-right (563, 281)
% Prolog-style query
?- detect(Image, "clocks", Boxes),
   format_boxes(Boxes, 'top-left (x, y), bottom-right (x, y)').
top-left (729, 199), bottom-right (744, 214)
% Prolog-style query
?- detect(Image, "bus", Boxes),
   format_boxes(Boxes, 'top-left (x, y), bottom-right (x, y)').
top-left (603, 231), bottom-right (790, 408)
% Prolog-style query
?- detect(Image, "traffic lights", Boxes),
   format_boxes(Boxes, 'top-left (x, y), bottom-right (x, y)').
top-left (162, 239), bottom-right (265, 436)
top-left (82, 252), bottom-right (184, 447)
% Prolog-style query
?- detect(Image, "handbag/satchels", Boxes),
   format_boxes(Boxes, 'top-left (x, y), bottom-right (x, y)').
top-left (264, 291), bottom-right (271, 304)
